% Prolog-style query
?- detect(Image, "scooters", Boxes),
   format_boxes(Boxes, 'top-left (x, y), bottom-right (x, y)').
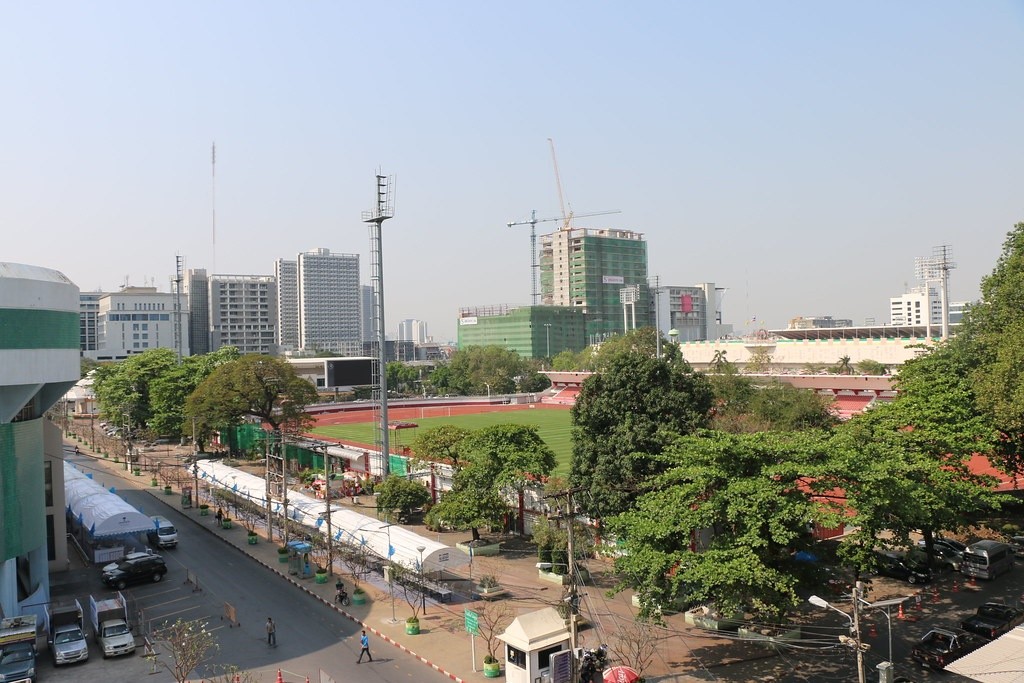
top-left (577, 643), bottom-right (609, 683)
top-left (335, 587), bottom-right (350, 606)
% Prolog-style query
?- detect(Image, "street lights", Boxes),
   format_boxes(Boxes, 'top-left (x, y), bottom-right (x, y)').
top-left (487, 384), bottom-right (490, 397)
top-left (356, 522), bottom-right (397, 620)
top-left (535, 560), bottom-right (580, 683)
top-left (840, 591), bottom-right (909, 683)
top-left (416, 546), bottom-right (427, 615)
top-left (422, 385), bottom-right (426, 399)
top-left (543, 323), bottom-right (553, 369)
top-left (231, 476), bottom-right (239, 520)
top-left (298, 486), bottom-right (334, 576)
top-left (807, 586), bottom-right (867, 683)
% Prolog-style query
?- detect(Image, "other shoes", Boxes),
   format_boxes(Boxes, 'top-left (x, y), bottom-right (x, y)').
top-left (356, 661), bottom-right (360, 664)
top-left (368, 659), bottom-right (372, 662)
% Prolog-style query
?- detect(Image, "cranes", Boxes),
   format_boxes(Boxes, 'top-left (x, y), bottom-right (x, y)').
top-left (506, 208), bottom-right (623, 306)
top-left (546, 136), bottom-right (574, 231)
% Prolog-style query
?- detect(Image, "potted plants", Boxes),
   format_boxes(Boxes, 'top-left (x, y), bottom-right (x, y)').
top-left (375, 556), bottom-right (434, 635)
top-left (472, 597), bottom-right (516, 678)
top-left (472, 558), bottom-right (505, 593)
top-left (67, 419), bottom-right (177, 495)
top-left (303, 530), bottom-right (340, 584)
top-left (276, 516), bottom-right (305, 563)
top-left (234, 502), bottom-right (268, 545)
top-left (329, 534), bottom-right (384, 605)
top-left (213, 488), bottom-right (234, 529)
top-left (198, 477), bottom-right (213, 516)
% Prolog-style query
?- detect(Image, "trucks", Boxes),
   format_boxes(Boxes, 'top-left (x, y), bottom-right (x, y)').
top-left (42, 598), bottom-right (90, 667)
top-left (88, 591), bottom-right (137, 660)
top-left (0, 613), bottom-right (40, 683)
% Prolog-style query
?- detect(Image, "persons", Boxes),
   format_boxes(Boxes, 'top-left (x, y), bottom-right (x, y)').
top-left (334, 578), bottom-right (344, 604)
top-left (217, 508), bottom-right (224, 527)
top-left (75, 445), bottom-right (79, 454)
top-left (356, 630), bottom-right (373, 664)
top-left (936, 634), bottom-right (946, 644)
top-left (265, 618), bottom-right (276, 646)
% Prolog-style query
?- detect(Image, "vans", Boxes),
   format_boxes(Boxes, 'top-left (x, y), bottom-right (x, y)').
top-left (150, 515), bottom-right (179, 548)
top-left (959, 539), bottom-right (1017, 581)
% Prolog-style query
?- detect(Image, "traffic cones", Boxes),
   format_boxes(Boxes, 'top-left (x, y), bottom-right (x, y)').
top-left (913, 595), bottom-right (924, 610)
top-left (969, 574), bottom-right (977, 586)
top-left (275, 668), bottom-right (283, 683)
top-left (952, 578), bottom-right (959, 593)
top-left (233, 673), bottom-right (240, 683)
top-left (896, 603), bottom-right (905, 619)
top-left (305, 676), bottom-right (310, 683)
top-left (933, 585), bottom-right (940, 602)
top-left (868, 625), bottom-right (878, 637)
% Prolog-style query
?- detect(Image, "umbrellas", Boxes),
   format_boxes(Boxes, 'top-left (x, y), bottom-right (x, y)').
top-left (602, 665), bottom-right (640, 683)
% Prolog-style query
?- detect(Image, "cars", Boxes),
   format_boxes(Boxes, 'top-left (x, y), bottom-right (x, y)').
top-left (960, 601), bottom-right (1024, 641)
top-left (866, 536), bottom-right (967, 586)
top-left (911, 624), bottom-right (984, 672)
top-left (101, 550), bottom-right (152, 574)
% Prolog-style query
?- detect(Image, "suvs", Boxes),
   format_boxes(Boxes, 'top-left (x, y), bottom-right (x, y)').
top-left (99, 554), bottom-right (168, 591)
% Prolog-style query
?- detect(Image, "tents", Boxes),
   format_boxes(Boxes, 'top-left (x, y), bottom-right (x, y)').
top-left (378, 420), bottom-right (418, 449)
